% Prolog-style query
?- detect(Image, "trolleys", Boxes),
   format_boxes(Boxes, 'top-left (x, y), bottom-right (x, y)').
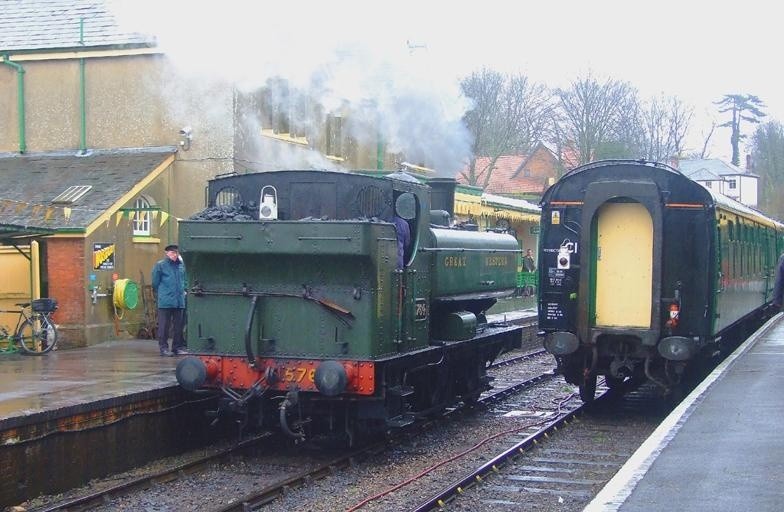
top-left (134, 266), bottom-right (161, 341)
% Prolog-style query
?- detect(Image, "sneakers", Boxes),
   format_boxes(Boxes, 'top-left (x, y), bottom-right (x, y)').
top-left (173, 349), bottom-right (187, 355)
top-left (160, 351), bottom-right (174, 356)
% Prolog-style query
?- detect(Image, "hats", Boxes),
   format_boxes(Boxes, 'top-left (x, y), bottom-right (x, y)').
top-left (165, 245), bottom-right (178, 251)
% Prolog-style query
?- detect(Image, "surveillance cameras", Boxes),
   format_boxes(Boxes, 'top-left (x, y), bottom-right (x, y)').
top-left (179, 127), bottom-right (193, 139)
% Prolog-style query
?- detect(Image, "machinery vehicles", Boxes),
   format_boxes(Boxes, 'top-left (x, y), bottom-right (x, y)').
top-left (174, 164), bottom-right (522, 451)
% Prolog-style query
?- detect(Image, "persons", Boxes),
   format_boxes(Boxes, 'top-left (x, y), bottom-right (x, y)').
top-left (177, 256), bottom-right (189, 349)
top-left (522, 249), bottom-right (536, 296)
top-left (151, 245), bottom-right (189, 355)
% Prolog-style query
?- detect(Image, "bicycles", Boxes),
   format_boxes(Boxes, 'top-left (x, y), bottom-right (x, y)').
top-left (0, 298), bottom-right (58, 356)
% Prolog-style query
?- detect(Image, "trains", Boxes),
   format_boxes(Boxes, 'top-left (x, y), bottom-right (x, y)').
top-left (534, 158), bottom-right (783, 407)
